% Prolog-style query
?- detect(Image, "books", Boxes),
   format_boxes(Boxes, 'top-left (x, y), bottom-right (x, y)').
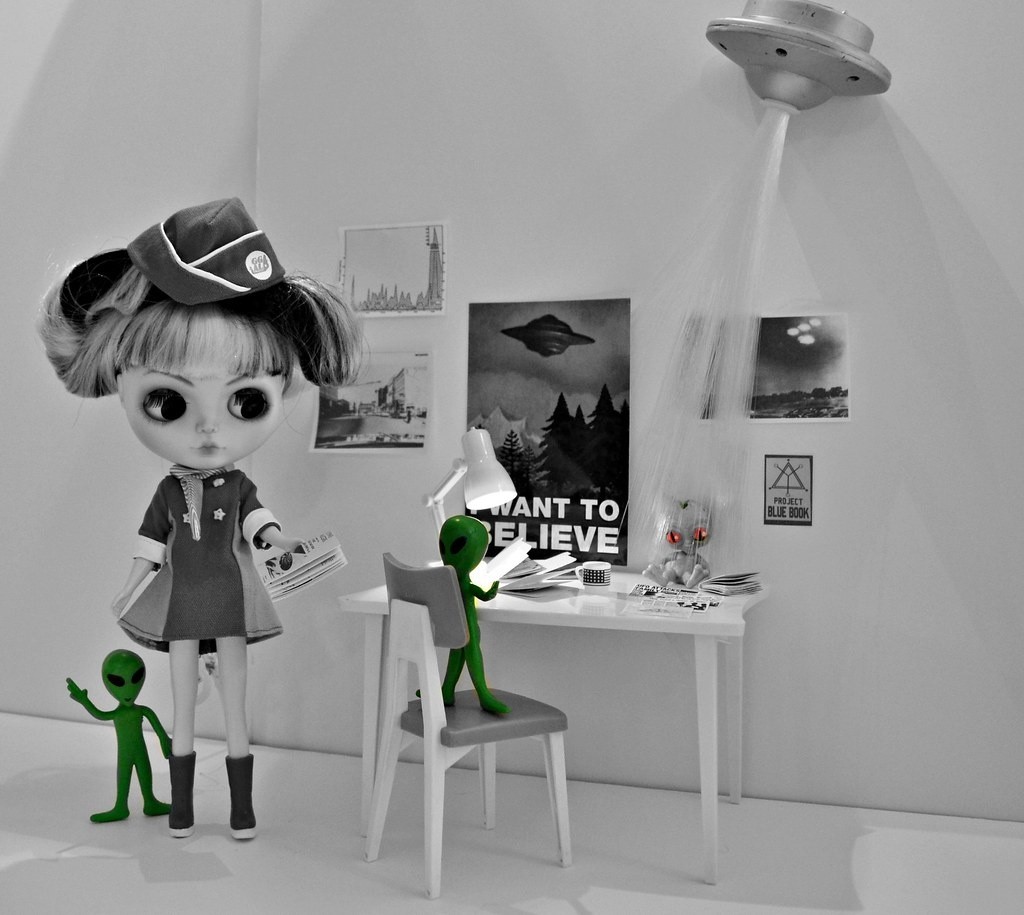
top-left (698, 571), bottom-right (764, 597)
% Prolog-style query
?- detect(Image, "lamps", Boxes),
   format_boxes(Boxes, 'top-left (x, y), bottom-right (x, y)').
top-left (423, 425), bottom-right (518, 561)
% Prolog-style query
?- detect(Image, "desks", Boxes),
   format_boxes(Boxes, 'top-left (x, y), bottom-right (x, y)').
top-left (337, 551), bottom-right (770, 891)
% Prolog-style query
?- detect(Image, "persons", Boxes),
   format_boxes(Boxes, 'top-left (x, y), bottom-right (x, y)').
top-left (35, 194), bottom-right (371, 841)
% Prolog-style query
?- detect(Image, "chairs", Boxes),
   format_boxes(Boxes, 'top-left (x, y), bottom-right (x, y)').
top-left (359, 552), bottom-right (575, 900)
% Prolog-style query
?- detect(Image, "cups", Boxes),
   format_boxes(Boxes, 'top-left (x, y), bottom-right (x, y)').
top-left (576, 561), bottom-right (612, 586)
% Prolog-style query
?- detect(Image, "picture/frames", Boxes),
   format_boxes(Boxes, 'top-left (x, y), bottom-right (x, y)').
top-left (746, 313), bottom-right (855, 423)
top-left (307, 342), bottom-right (441, 457)
top-left (461, 296), bottom-right (637, 567)
top-left (335, 221), bottom-right (450, 322)
